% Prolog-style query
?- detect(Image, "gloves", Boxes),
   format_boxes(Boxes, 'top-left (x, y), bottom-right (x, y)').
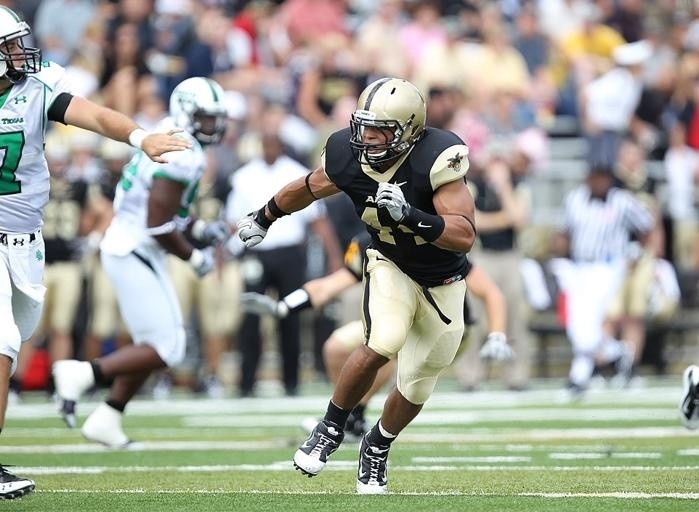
top-left (236, 285), bottom-right (313, 321)
top-left (189, 218), bottom-right (230, 248)
top-left (236, 203), bottom-right (277, 249)
top-left (188, 249), bottom-right (214, 279)
top-left (477, 331), bottom-right (515, 363)
top-left (374, 180), bottom-right (445, 244)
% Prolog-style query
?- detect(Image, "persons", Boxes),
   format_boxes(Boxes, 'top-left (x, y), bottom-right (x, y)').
top-left (48, 72), bottom-right (240, 450)
top-left (1, 0), bottom-right (699, 427)
top-left (236, 230), bottom-right (475, 445)
top-left (0, 0), bottom-right (197, 502)
top-left (234, 76), bottom-right (482, 496)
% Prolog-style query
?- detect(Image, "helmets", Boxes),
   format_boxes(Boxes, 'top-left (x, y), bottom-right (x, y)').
top-left (169, 77), bottom-right (229, 145)
top-left (0, 5), bottom-right (43, 80)
top-left (349, 76), bottom-right (427, 168)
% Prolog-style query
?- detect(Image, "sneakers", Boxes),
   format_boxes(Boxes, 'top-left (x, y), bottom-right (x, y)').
top-left (0, 464), bottom-right (36, 501)
top-left (300, 406), bottom-right (372, 444)
top-left (355, 430), bottom-right (390, 496)
top-left (81, 405), bottom-right (133, 451)
top-left (678, 363), bottom-right (699, 431)
top-left (49, 358), bottom-right (91, 430)
top-left (291, 418), bottom-right (346, 478)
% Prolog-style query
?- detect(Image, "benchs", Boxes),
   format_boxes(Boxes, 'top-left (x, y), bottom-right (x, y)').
top-left (528, 315), bottom-right (694, 381)
